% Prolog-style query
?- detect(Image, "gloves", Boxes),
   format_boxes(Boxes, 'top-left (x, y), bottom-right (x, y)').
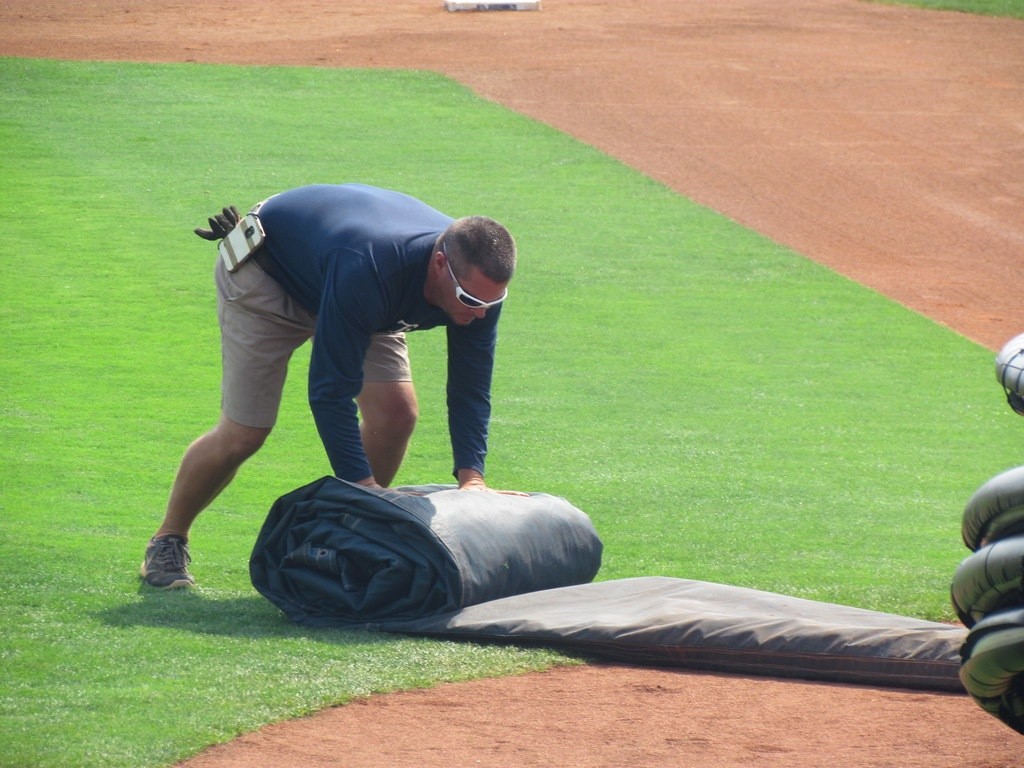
top-left (194, 205), bottom-right (240, 242)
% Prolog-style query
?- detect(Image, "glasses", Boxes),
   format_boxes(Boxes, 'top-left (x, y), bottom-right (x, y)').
top-left (443, 242), bottom-right (508, 310)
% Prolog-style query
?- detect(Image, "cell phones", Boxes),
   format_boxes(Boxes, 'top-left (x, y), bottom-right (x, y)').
top-left (219, 213), bottom-right (265, 273)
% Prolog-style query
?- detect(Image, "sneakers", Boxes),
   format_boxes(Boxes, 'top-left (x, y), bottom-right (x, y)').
top-left (139, 531), bottom-right (196, 590)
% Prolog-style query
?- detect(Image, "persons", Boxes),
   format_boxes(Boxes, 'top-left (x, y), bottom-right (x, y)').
top-left (138, 176), bottom-right (517, 589)
